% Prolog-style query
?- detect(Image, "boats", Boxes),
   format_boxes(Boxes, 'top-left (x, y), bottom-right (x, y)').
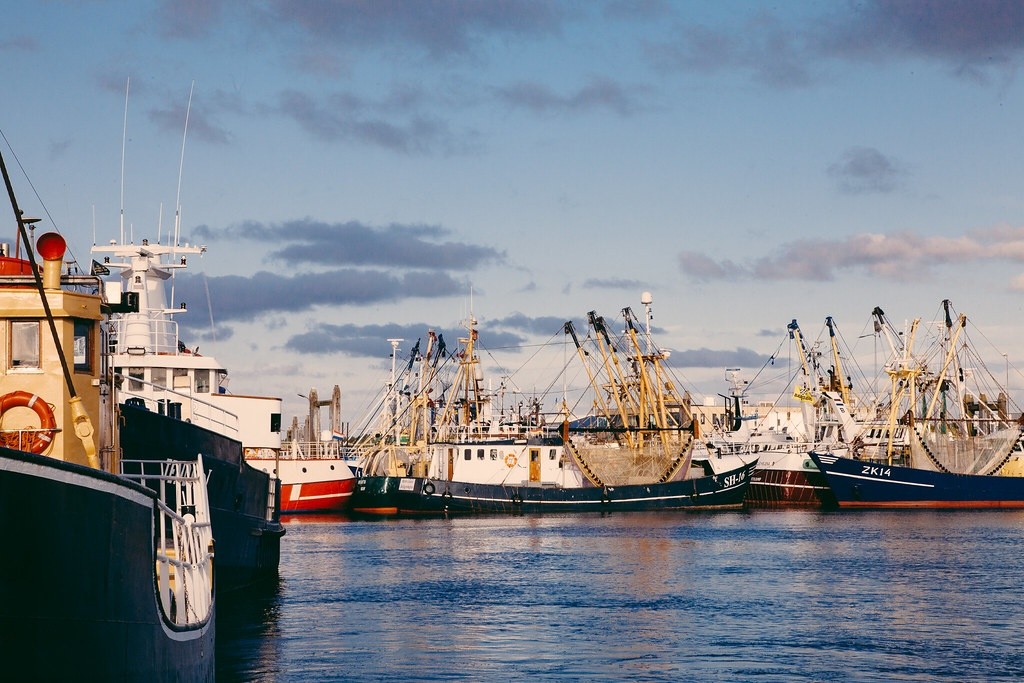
top-left (1, 75), bottom-right (1024, 683)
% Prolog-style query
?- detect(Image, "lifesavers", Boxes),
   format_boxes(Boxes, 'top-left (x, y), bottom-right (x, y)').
top-left (505, 454), bottom-right (518, 467)
top-left (0, 391), bottom-right (56, 455)
